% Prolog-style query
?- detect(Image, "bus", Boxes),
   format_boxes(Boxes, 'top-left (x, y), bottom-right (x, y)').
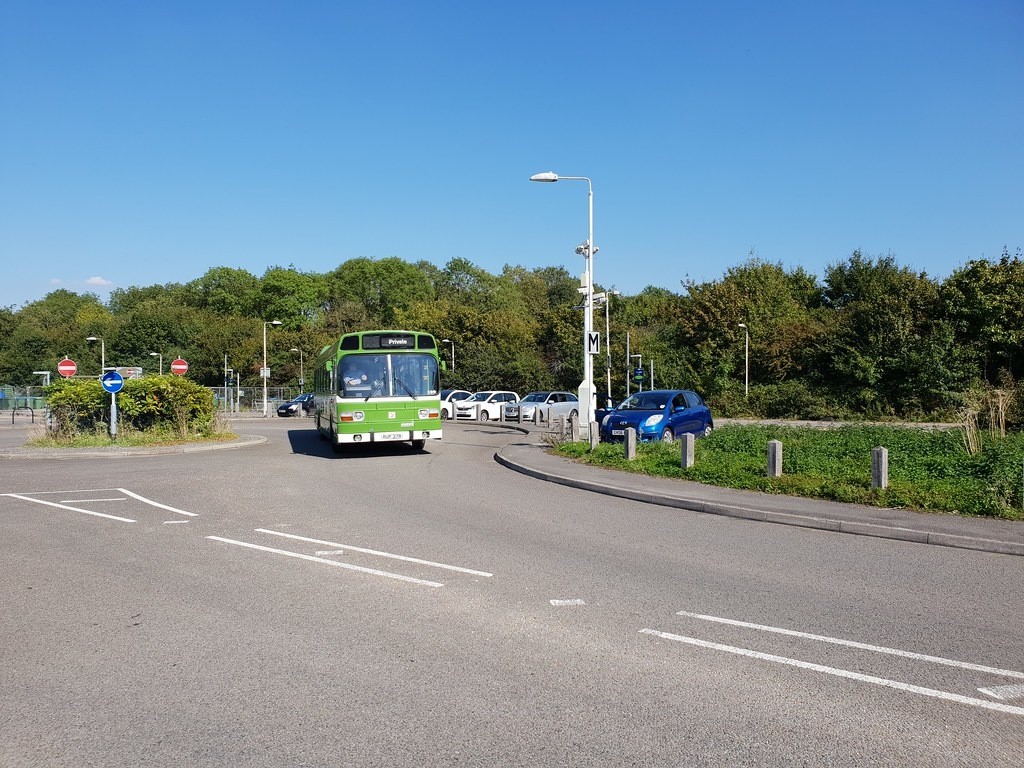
top-left (312, 329), bottom-right (444, 452)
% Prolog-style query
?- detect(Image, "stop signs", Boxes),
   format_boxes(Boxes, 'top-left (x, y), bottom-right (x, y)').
top-left (57, 354), bottom-right (77, 379)
top-left (171, 355), bottom-right (189, 376)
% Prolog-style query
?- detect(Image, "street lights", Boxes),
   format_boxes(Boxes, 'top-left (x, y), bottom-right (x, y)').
top-left (442, 338), bottom-right (455, 374)
top-left (86, 337), bottom-right (106, 377)
top-left (260, 320), bottom-right (283, 417)
top-left (528, 170), bottom-right (599, 445)
top-left (737, 322), bottom-right (749, 400)
top-left (149, 352), bottom-right (164, 376)
top-left (291, 348), bottom-right (304, 395)
top-left (605, 287), bottom-right (621, 409)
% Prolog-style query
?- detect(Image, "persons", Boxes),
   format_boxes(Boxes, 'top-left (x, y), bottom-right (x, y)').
top-left (343, 362), bottom-right (368, 386)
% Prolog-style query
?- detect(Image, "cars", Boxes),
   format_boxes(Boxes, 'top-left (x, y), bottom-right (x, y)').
top-left (278, 392), bottom-right (313, 418)
top-left (454, 388), bottom-right (521, 420)
top-left (439, 388), bottom-right (474, 420)
top-left (597, 388), bottom-right (715, 448)
top-left (504, 387), bottom-right (580, 424)
